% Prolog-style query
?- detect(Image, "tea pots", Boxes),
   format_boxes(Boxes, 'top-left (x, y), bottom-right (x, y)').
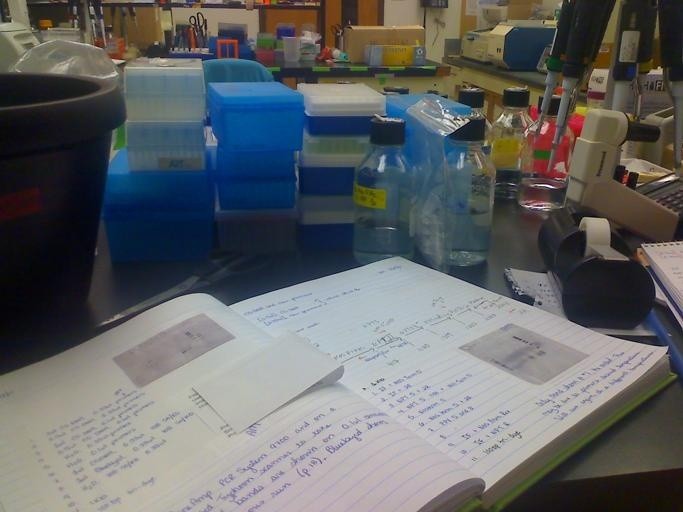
top-left (281, 36), bottom-right (302, 62)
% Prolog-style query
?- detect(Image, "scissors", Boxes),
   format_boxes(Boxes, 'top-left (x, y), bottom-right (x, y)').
top-left (189, 12), bottom-right (204, 29)
top-left (331, 23), bottom-right (343, 33)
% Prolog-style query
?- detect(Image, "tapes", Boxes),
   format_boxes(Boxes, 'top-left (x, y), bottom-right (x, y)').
top-left (578, 216), bottom-right (628, 259)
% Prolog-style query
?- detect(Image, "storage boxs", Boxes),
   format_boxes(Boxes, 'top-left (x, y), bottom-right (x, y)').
top-left (298, 84), bottom-right (388, 136)
top-left (386, 94), bottom-right (470, 163)
top-left (123, 51), bottom-right (206, 122)
top-left (105, 147), bottom-right (214, 263)
top-left (126, 121), bottom-right (205, 172)
top-left (297, 139), bottom-right (368, 196)
top-left (217, 150), bottom-right (296, 210)
top-left (345, 24), bottom-right (425, 63)
top-left (299, 210), bottom-right (355, 249)
top-left (208, 82), bottom-right (304, 150)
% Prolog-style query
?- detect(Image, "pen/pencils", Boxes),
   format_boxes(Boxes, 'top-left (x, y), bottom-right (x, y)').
top-left (643, 309), bottom-right (683, 379)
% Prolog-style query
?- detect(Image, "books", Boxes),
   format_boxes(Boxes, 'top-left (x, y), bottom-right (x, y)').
top-left (502, 266), bottom-right (659, 343)
top-left (0, 256), bottom-right (677, 512)
top-left (635, 241), bottom-right (683, 335)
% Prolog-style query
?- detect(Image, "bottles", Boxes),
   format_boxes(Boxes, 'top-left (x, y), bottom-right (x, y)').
top-left (443, 116), bottom-right (497, 269)
top-left (350, 114), bottom-right (415, 266)
top-left (490, 86), bottom-right (536, 201)
top-left (457, 87), bottom-right (494, 165)
top-left (517, 93), bottom-right (575, 214)
